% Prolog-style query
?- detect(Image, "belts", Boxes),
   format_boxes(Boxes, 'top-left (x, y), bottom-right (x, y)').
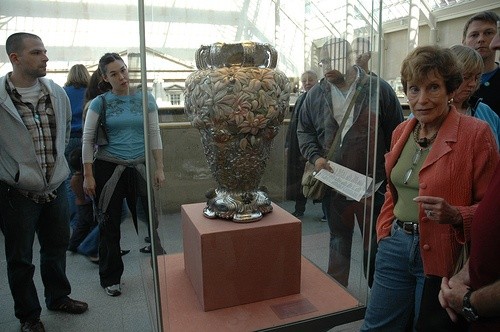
top-left (396, 220), bottom-right (418, 236)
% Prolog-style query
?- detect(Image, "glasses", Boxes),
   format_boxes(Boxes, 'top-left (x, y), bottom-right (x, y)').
top-left (318, 57), bottom-right (344, 67)
top-left (403, 147), bottom-right (422, 185)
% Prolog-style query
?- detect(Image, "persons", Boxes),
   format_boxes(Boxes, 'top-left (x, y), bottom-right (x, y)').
top-left (460, 12), bottom-right (500, 111)
top-left (286, 71), bottom-right (327, 224)
top-left (297, 38), bottom-right (405, 291)
top-left (75, 67), bottom-right (130, 260)
top-left (81, 52), bottom-right (165, 298)
top-left (350, 39), bottom-right (378, 76)
top-left (447, 45), bottom-right (500, 142)
top-left (61, 63), bottom-right (96, 249)
top-left (362, 46), bottom-right (500, 331)
top-left (0, 32), bottom-right (88, 331)
top-left (489, 31), bottom-right (500, 56)
top-left (438, 159), bottom-right (500, 329)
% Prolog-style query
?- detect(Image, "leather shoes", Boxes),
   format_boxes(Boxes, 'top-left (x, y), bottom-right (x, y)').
top-left (46, 297), bottom-right (88, 314)
top-left (78, 246), bottom-right (99, 265)
top-left (20, 319), bottom-right (45, 332)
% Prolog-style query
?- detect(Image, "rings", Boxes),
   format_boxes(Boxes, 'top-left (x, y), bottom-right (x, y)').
top-left (426, 210), bottom-right (433, 217)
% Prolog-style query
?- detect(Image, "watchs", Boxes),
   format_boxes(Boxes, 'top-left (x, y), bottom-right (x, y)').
top-left (459, 289), bottom-right (482, 329)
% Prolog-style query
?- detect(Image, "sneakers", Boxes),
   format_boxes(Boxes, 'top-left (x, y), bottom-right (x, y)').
top-left (104, 284), bottom-right (121, 296)
top-left (293, 212), bottom-right (304, 217)
top-left (321, 216), bottom-right (328, 222)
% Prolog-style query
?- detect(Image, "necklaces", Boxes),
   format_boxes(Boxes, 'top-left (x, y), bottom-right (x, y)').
top-left (415, 123), bottom-right (440, 146)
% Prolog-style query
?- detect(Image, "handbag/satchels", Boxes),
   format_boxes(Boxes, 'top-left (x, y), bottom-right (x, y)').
top-left (300, 73), bottom-right (369, 200)
top-left (94, 95), bottom-right (109, 147)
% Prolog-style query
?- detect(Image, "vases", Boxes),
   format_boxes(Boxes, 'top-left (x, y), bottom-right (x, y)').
top-left (184, 43), bottom-right (290, 223)
